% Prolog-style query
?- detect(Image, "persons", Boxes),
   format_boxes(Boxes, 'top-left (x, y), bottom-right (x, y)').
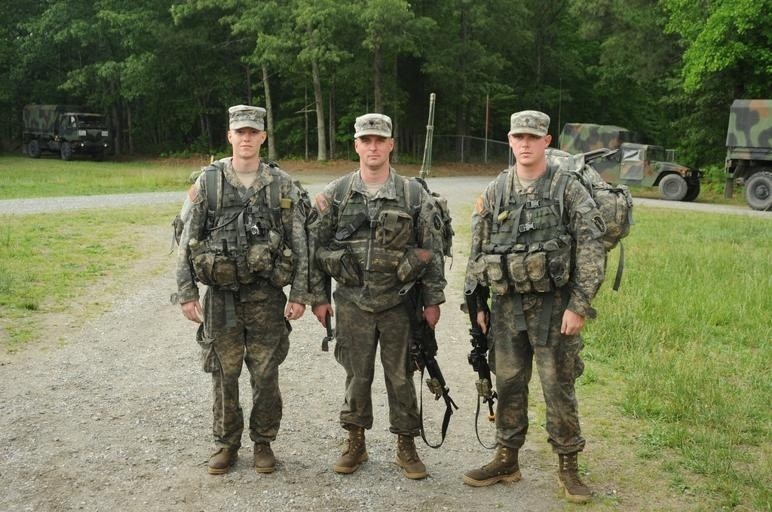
top-left (461, 110), bottom-right (608, 502)
top-left (175, 104), bottom-right (311, 473)
top-left (307, 113), bottom-right (447, 479)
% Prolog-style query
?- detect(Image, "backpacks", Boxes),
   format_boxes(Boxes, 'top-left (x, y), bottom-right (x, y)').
top-left (411, 177), bottom-right (455, 257)
top-left (545, 148), bottom-right (634, 249)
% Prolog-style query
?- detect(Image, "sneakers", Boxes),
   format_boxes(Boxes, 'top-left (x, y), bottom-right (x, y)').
top-left (253, 443), bottom-right (275, 472)
top-left (208, 449), bottom-right (238, 473)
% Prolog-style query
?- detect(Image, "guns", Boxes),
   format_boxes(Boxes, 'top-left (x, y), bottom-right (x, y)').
top-left (466, 278), bottom-right (497, 417)
top-left (399, 280), bottom-right (458, 416)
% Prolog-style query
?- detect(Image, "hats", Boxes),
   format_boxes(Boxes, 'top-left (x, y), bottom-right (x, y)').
top-left (354, 114), bottom-right (393, 137)
top-left (509, 110), bottom-right (550, 137)
top-left (228, 105), bottom-right (267, 131)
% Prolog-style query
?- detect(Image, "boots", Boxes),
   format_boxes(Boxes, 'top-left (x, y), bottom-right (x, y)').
top-left (558, 452), bottom-right (591, 502)
top-left (395, 433), bottom-right (427, 478)
top-left (333, 425), bottom-right (368, 473)
top-left (464, 444), bottom-right (521, 486)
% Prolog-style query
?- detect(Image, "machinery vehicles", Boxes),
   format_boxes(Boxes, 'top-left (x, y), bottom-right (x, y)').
top-left (722, 98), bottom-right (772, 211)
top-left (558, 122), bottom-right (703, 203)
top-left (18, 102), bottom-right (113, 163)
